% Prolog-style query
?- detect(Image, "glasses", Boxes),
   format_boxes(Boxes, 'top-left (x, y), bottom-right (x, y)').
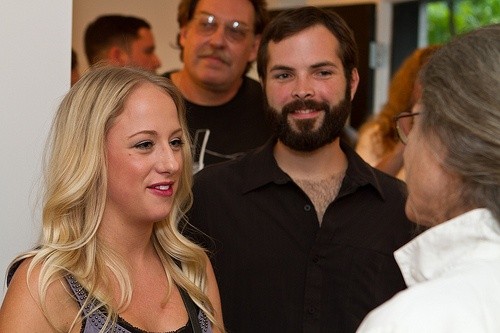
top-left (191, 12), bottom-right (255, 41)
top-left (393, 110), bottom-right (426, 145)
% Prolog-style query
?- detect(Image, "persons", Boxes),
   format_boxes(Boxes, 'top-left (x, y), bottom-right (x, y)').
top-left (0, 63), bottom-right (225, 333)
top-left (72, 0), bottom-right (275, 171)
top-left (177, 8), bottom-right (430, 333)
top-left (356, 24), bottom-right (499, 333)
